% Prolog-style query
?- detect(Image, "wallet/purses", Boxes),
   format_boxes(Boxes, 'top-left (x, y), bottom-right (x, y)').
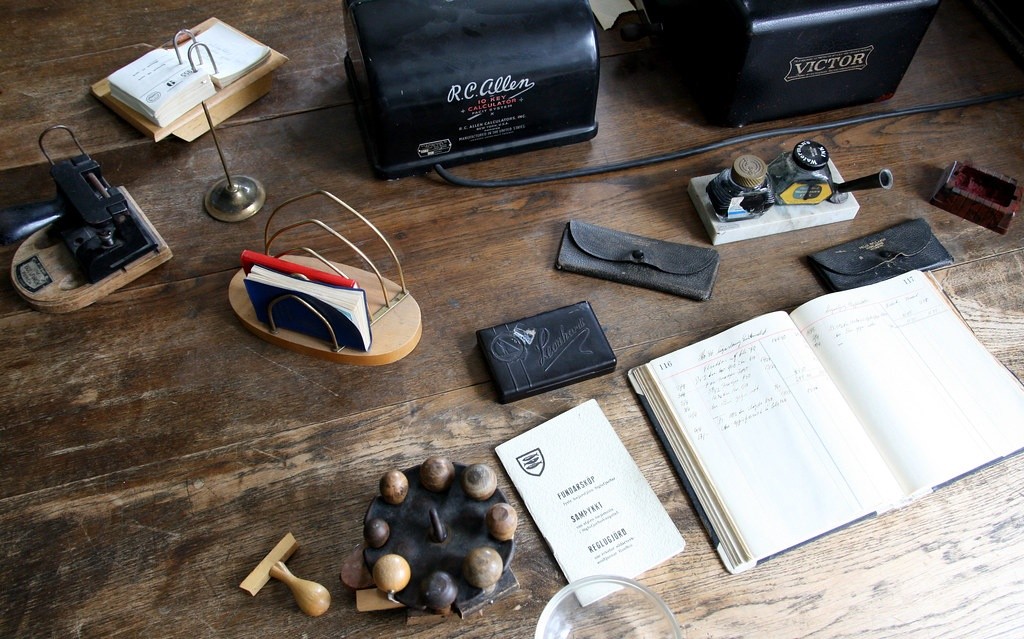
top-left (807, 217), bottom-right (954, 291)
top-left (556, 220), bottom-right (722, 299)
top-left (476, 300), bottom-right (619, 404)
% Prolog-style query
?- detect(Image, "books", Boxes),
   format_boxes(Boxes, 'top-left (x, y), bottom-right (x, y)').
top-left (627, 267), bottom-right (1024, 576)
top-left (243, 263), bottom-right (373, 353)
top-left (239, 249), bottom-right (360, 290)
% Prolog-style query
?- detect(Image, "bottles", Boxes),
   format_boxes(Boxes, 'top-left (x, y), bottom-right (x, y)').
top-left (768, 140), bottom-right (834, 206)
top-left (706, 156), bottom-right (774, 222)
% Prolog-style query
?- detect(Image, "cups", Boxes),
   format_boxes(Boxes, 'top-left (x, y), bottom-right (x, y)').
top-left (532, 575), bottom-right (682, 639)
